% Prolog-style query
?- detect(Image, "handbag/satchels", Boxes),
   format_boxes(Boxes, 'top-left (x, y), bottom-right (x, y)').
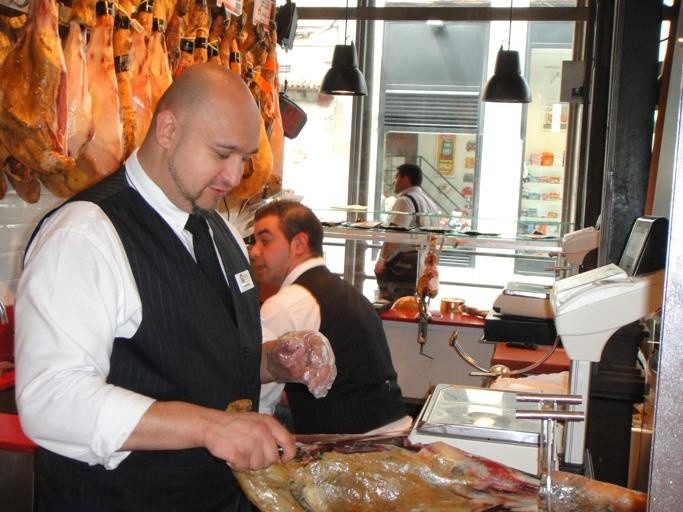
top-left (376, 251), bottom-right (417, 302)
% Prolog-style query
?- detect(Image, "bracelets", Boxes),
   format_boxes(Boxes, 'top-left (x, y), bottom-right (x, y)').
top-left (378, 257), bottom-right (385, 264)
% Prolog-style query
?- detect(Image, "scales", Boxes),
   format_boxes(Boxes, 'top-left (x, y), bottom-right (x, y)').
top-left (492, 227), bottom-right (600, 319)
top-left (403, 215), bottom-right (669, 476)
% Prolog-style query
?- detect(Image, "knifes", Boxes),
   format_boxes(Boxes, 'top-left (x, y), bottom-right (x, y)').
top-left (272, 433), bottom-right (410, 456)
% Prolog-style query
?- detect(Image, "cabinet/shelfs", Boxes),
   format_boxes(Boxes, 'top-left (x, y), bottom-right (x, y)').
top-left (314, 209), bottom-right (562, 407)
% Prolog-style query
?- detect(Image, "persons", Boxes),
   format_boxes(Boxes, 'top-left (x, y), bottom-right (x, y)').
top-left (374, 163), bottom-right (437, 302)
top-left (249, 199), bottom-right (414, 437)
top-left (14, 64), bottom-right (337, 512)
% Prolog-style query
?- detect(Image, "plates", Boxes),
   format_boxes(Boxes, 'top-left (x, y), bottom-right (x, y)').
top-left (320, 220), bottom-right (557, 239)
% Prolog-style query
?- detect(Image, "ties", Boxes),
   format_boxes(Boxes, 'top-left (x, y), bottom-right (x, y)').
top-left (183, 214), bottom-right (237, 323)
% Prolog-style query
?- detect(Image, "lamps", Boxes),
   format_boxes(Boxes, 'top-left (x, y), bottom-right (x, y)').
top-left (316, 0), bottom-right (367, 98)
top-left (477, 1), bottom-right (532, 106)
